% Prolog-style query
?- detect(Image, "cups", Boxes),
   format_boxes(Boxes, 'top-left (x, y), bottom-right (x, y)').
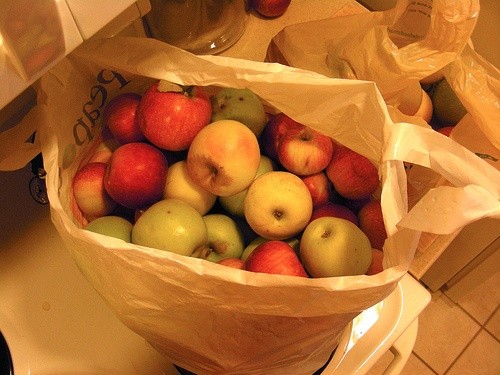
top-left (146, 0), bottom-right (249, 55)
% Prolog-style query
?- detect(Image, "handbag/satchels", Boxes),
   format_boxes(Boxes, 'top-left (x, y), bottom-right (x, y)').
top-left (265, 0), bottom-right (500, 176)
top-left (36, 38), bottom-right (500, 375)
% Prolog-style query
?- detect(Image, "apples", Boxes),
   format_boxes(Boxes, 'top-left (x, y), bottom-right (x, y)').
top-left (71, 77), bottom-right (387, 279)
top-left (410, 70), bottom-right (468, 137)
